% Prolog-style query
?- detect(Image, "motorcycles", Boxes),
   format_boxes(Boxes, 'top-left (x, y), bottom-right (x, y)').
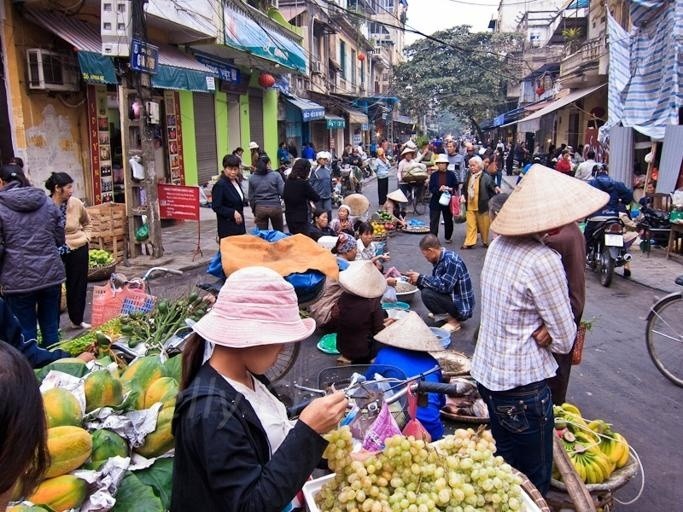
top-left (336, 164), bottom-right (362, 197)
top-left (585, 217), bottom-right (631, 287)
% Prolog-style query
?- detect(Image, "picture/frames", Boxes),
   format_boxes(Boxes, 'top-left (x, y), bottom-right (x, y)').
top-left (167, 115), bottom-right (177, 128)
top-left (169, 142), bottom-right (178, 156)
top-left (170, 156), bottom-right (179, 169)
top-left (97, 118), bottom-right (111, 132)
top-left (95, 85), bottom-right (107, 96)
top-left (101, 192), bottom-right (115, 203)
top-left (100, 177), bottom-right (113, 193)
top-left (99, 161), bottom-right (112, 178)
top-left (98, 132), bottom-right (110, 146)
top-left (99, 146), bottom-right (112, 161)
top-left (171, 169), bottom-right (181, 181)
top-left (96, 96), bottom-right (108, 118)
top-left (164, 96), bottom-right (175, 115)
top-left (172, 179), bottom-right (181, 185)
top-left (139, 72), bottom-right (151, 88)
top-left (168, 128), bottom-right (177, 141)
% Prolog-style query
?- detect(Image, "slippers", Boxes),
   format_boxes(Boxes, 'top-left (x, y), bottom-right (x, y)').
top-left (427, 311), bottom-right (449, 322)
top-left (439, 324), bottom-right (461, 335)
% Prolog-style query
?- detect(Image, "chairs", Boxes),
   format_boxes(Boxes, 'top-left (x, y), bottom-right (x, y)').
top-left (643, 193), bottom-right (673, 259)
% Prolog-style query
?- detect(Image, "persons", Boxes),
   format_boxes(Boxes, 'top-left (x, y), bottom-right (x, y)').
top-left (332, 260), bottom-right (397, 364)
top-left (574, 151), bottom-right (602, 181)
top-left (355, 222), bottom-right (391, 274)
top-left (302, 143), bottom-right (314, 159)
top-left (395, 148), bottom-right (428, 210)
top-left (584, 164), bottom-right (634, 276)
top-left (0, 166), bottom-right (66, 348)
top-left (232, 147), bottom-right (249, 173)
top-left (277, 141), bottom-right (289, 165)
top-left (0, 298), bottom-right (95, 369)
top-left (363, 311), bottom-right (446, 442)
top-left (556, 150), bottom-right (571, 174)
top-left (329, 204), bottom-right (354, 236)
top-left (407, 234), bottom-right (475, 332)
top-left (543, 222), bottom-right (586, 407)
top-left (211, 155), bottom-right (246, 239)
top-left (170, 265), bottom-right (348, 512)
top-left (469, 164), bottom-right (577, 498)
top-left (309, 208), bottom-right (335, 242)
top-left (639, 181), bottom-right (657, 207)
top-left (382, 189), bottom-right (408, 231)
top-left (309, 151), bottom-right (332, 212)
top-left (46, 171), bottom-right (92, 330)
top-left (250, 142), bottom-right (260, 167)
top-left (373, 148), bottom-right (391, 207)
top-left (311, 233), bottom-right (396, 333)
top-left (248, 153), bottom-right (285, 232)
top-left (428, 153), bottom-right (459, 243)
top-left (460, 156), bottom-right (501, 250)
top-left (0, 338), bottom-right (51, 512)
top-left (377, 128), bottom-right (583, 163)
top-left (284, 160), bottom-right (320, 236)
top-left (348, 148), bottom-right (363, 194)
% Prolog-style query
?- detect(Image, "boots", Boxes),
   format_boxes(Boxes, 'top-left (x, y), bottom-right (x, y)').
top-left (434, 153), bottom-right (449, 167)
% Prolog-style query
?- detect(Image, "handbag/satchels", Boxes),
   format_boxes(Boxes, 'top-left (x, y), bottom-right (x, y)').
top-left (454, 187), bottom-right (468, 225)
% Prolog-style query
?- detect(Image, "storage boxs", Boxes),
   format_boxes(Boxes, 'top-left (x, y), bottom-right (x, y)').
top-left (87, 203), bottom-right (127, 237)
top-left (87, 237), bottom-right (128, 264)
top-left (302, 436), bottom-right (542, 512)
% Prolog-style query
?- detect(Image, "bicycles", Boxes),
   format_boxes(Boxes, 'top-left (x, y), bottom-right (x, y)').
top-left (92, 268), bottom-right (300, 386)
top-left (285, 364), bottom-right (457, 420)
top-left (403, 163), bottom-right (435, 215)
top-left (640, 277), bottom-right (683, 389)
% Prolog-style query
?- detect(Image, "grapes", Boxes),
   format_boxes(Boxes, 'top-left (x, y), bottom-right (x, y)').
top-left (314, 425), bottom-right (524, 512)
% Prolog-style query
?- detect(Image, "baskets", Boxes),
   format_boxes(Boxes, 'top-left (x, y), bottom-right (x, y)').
top-left (571, 320), bottom-right (587, 366)
top-left (437, 408), bottom-right (495, 424)
top-left (86, 264), bottom-right (122, 283)
top-left (430, 352), bottom-right (472, 380)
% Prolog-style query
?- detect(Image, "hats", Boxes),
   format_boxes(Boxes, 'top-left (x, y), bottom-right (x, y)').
top-left (489, 162), bottom-right (611, 238)
top-left (386, 188), bottom-right (409, 203)
top-left (400, 148), bottom-right (415, 156)
top-left (338, 259), bottom-right (388, 299)
top-left (344, 194), bottom-right (368, 216)
top-left (373, 311), bottom-right (446, 355)
top-left (315, 151), bottom-right (328, 162)
top-left (191, 267), bottom-right (316, 350)
top-left (377, 148), bottom-right (385, 155)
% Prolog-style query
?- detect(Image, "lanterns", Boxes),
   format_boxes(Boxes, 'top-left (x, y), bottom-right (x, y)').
top-left (535, 87), bottom-right (545, 97)
top-left (257, 73), bottom-right (275, 90)
top-left (357, 52), bottom-right (365, 61)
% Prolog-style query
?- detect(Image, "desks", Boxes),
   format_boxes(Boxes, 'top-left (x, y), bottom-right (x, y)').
top-left (666, 224), bottom-right (683, 265)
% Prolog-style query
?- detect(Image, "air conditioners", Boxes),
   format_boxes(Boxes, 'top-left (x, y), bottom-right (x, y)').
top-left (26, 47), bottom-right (81, 93)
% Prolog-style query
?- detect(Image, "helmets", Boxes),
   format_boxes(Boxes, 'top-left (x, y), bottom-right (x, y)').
top-left (591, 162), bottom-right (610, 177)
top-left (249, 141), bottom-right (259, 151)
top-left (466, 144), bottom-right (476, 152)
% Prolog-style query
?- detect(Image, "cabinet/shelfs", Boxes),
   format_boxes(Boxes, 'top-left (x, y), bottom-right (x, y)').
top-left (95, 328), bottom-right (184, 361)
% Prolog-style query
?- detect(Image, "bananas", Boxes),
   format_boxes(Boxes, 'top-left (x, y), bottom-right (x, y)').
top-left (559, 432), bottom-right (600, 452)
top-left (553, 445), bottom-right (611, 484)
top-left (554, 402), bottom-right (587, 432)
top-left (588, 419), bottom-right (629, 471)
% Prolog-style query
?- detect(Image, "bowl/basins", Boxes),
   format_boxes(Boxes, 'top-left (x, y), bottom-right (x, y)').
top-left (442, 339), bottom-right (451, 348)
top-left (428, 327), bottom-right (450, 343)
top-left (396, 281), bottom-right (419, 302)
top-left (382, 301), bottom-right (410, 311)
